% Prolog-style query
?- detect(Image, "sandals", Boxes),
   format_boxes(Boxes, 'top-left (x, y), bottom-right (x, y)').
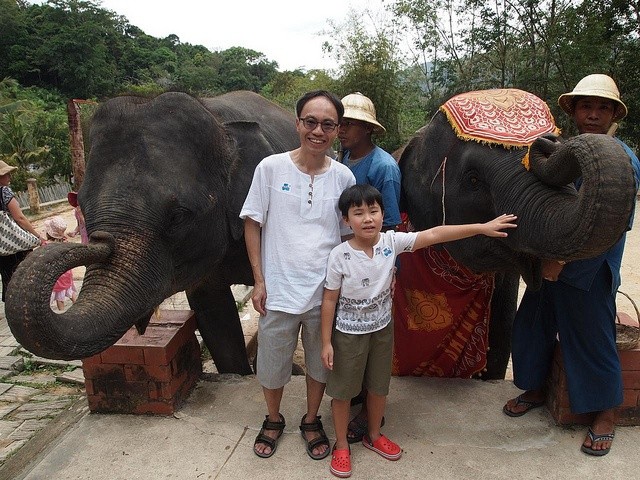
top-left (330, 442), bottom-right (352, 477)
top-left (299, 414), bottom-right (330, 460)
top-left (363, 432), bottom-right (402, 461)
top-left (254, 413), bottom-right (286, 458)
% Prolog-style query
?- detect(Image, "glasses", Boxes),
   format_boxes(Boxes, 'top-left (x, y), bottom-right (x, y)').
top-left (299, 117), bottom-right (339, 133)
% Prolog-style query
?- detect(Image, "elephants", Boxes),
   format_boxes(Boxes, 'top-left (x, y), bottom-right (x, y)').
top-left (5, 89), bottom-right (300, 374)
top-left (393, 89), bottom-right (636, 380)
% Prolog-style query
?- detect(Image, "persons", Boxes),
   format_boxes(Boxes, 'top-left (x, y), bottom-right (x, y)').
top-left (504, 74), bottom-right (640, 457)
top-left (42, 216), bottom-right (76, 310)
top-left (321, 185), bottom-right (518, 478)
top-left (0, 160), bottom-right (45, 302)
top-left (337, 92), bottom-right (403, 442)
top-left (68, 192), bottom-right (90, 244)
top-left (240, 95), bottom-right (357, 459)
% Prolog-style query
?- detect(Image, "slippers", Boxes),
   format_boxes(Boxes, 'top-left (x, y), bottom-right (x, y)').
top-left (346, 416), bottom-right (384, 443)
top-left (581, 427), bottom-right (615, 455)
top-left (504, 394), bottom-right (544, 417)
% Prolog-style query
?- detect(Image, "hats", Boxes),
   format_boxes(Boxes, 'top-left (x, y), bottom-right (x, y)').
top-left (557, 74), bottom-right (628, 122)
top-left (341, 92), bottom-right (386, 134)
top-left (68, 192), bottom-right (78, 207)
top-left (0, 160), bottom-right (18, 176)
top-left (44, 216), bottom-right (66, 239)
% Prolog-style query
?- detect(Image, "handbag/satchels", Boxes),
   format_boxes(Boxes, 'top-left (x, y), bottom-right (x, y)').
top-left (0, 187), bottom-right (40, 256)
